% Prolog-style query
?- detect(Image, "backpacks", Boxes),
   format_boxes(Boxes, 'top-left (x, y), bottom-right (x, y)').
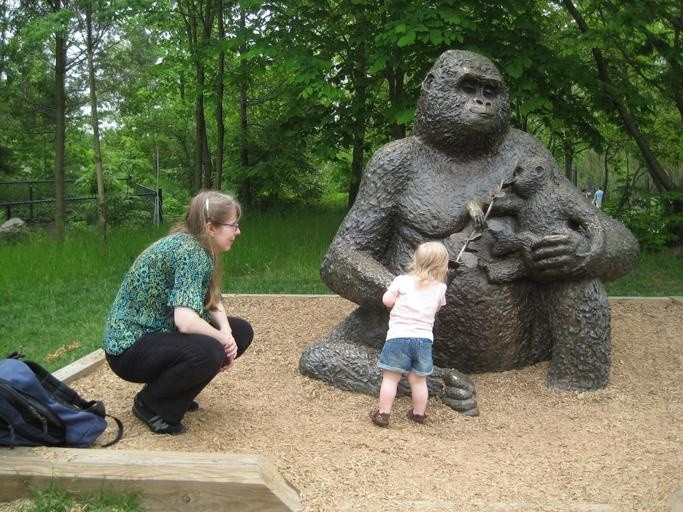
top-left (1, 351), bottom-right (124, 456)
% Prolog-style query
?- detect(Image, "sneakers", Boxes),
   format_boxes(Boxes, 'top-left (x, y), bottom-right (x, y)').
top-left (406, 408), bottom-right (427, 425)
top-left (370, 409), bottom-right (391, 427)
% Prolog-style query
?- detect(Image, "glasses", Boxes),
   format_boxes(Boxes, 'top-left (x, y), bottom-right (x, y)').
top-left (218, 223), bottom-right (239, 231)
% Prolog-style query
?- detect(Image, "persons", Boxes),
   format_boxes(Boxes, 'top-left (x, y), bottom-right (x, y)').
top-left (580, 189), bottom-right (588, 199)
top-left (368, 241), bottom-right (449, 428)
top-left (102, 190), bottom-right (254, 435)
top-left (594, 187), bottom-right (604, 208)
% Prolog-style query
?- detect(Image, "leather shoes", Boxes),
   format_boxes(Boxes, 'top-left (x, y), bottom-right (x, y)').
top-left (132, 394), bottom-right (187, 435)
top-left (189, 402), bottom-right (199, 412)
